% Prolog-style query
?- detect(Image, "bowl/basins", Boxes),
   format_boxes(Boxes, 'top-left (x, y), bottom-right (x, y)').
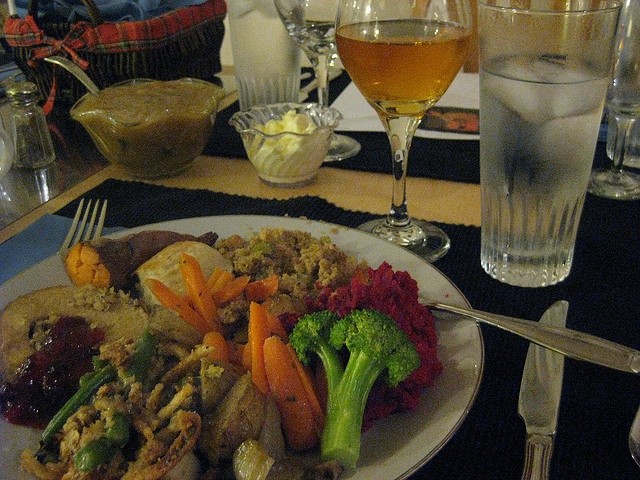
top-left (70, 78), bottom-right (238, 180)
top-left (228, 103), bottom-right (342, 187)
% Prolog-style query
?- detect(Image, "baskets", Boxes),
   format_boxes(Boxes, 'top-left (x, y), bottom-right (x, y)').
top-left (0, 0), bottom-right (227, 103)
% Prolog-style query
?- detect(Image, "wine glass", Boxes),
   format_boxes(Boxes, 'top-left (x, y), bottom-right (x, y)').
top-left (334, 0), bottom-right (471, 263)
top-left (587, 0), bottom-right (639, 202)
top-left (274, 0), bottom-right (362, 164)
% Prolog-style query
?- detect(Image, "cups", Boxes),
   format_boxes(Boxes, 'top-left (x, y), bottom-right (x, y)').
top-left (226, 1), bottom-right (304, 113)
top-left (476, 0), bottom-right (620, 288)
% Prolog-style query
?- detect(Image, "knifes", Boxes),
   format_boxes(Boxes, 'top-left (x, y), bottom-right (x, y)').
top-left (518, 300), bottom-right (569, 479)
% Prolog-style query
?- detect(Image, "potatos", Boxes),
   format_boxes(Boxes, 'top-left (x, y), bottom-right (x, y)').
top-left (63, 230), bottom-right (219, 290)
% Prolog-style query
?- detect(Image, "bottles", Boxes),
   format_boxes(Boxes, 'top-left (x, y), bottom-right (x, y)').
top-left (5, 82), bottom-right (56, 169)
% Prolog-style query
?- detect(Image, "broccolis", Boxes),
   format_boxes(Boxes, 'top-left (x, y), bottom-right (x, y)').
top-left (288, 311), bottom-right (420, 479)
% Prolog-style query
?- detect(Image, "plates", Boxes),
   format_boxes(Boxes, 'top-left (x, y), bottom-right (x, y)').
top-left (1, 215), bottom-right (487, 479)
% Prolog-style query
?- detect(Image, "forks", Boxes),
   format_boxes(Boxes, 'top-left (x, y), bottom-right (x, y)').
top-left (57, 199), bottom-right (108, 253)
